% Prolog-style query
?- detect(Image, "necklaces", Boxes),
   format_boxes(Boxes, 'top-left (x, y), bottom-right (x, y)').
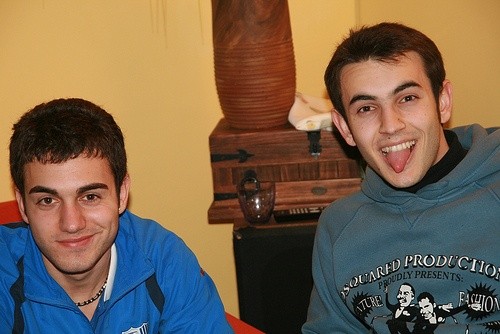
top-left (75, 248), bottom-right (111, 307)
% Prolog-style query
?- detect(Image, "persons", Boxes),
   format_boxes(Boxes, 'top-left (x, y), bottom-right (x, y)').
top-left (0, 98), bottom-right (235, 334)
top-left (301, 22), bottom-right (500, 334)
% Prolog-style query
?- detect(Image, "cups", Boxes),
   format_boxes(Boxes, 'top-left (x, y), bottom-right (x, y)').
top-left (236, 183), bottom-right (275, 225)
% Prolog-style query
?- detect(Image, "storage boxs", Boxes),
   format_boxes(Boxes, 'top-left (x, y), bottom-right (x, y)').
top-left (233, 220), bottom-right (318, 334)
top-left (209, 117), bottom-right (365, 204)
